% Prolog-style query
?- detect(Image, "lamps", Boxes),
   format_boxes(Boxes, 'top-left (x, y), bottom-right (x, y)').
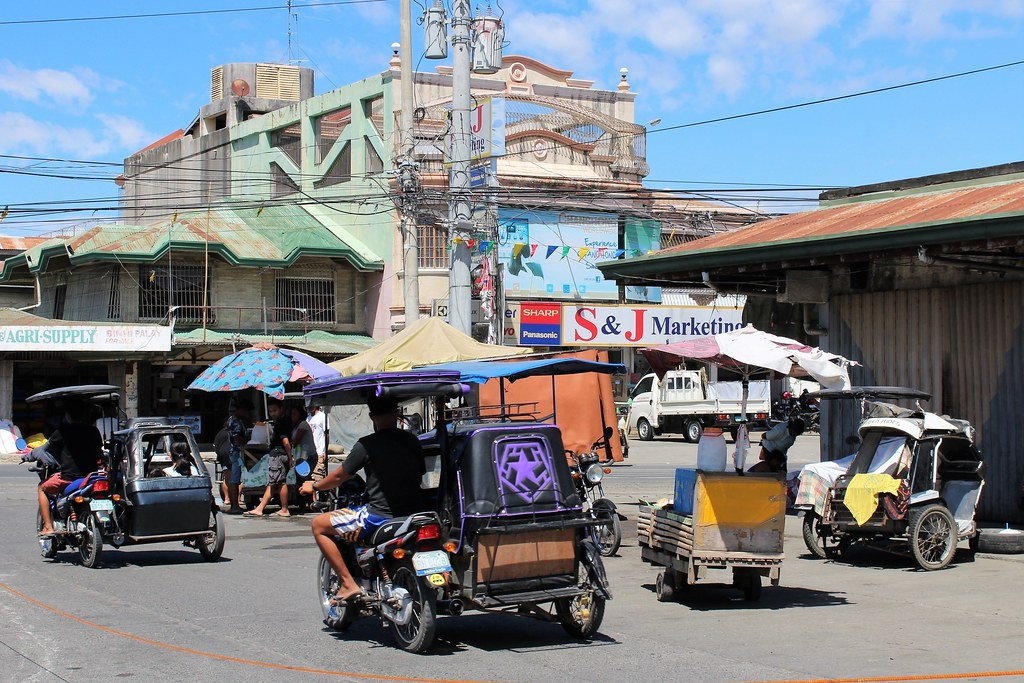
top-left (644, 118), bottom-right (661, 127)
top-left (391, 42), bottom-right (400, 54)
top-left (620, 67), bottom-right (629, 77)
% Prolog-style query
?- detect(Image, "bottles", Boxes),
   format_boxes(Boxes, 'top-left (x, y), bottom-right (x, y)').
top-left (696, 428), bottom-right (727, 472)
top-left (251, 423), bottom-right (267, 444)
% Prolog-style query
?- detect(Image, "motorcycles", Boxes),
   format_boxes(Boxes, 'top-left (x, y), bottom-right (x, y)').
top-left (803, 384), bottom-right (987, 571)
top-left (16, 386), bottom-right (228, 568)
top-left (765, 389), bottom-right (821, 432)
top-left (564, 427), bottom-right (621, 557)
top-left (294, 368), bottom-right (609, 654)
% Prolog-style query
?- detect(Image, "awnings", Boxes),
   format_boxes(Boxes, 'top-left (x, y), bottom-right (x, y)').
top-left (324, 314), bottom-right (534, 378)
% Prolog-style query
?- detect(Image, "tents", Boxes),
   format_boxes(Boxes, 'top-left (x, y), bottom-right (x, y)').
top-left (172, 326), bottom-right (383, 350)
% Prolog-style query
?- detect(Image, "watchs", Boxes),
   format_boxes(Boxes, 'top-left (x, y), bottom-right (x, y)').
top-left (312, 479), bottom-right (320, 491)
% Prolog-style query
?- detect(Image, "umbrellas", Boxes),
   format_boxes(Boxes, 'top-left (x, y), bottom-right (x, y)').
top-left (637, 321), bottom-right (865, 476)
top-left (183, 341), bottom-right (344, 423)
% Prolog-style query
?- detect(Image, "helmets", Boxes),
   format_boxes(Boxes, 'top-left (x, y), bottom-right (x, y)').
top-left (783, 391), bottom-right (792, 400)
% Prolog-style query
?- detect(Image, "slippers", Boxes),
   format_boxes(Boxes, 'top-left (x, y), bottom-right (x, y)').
top-left (269, 512), bottom-right (292, 519)
top-left (242, 511), bottom-right (264, 517)
top-left (331, 591), bottom-right (362, 608)
top-left (37, 532), bottom-right (56, 537)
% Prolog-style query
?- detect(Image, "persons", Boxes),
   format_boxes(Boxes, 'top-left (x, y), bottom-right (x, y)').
top-left (147, 439), bottom-right (200, 477)
top-left (297, 396), bottom-right (428, 606)
top-left (20, 399), bottom-right (106, 537)
top-left (748, 418), bottom-right (804, 474)
top-left (213, 396), bottom-right (331, 516)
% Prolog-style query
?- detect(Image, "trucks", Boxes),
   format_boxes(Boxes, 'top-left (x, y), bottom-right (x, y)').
top-left (627, 370), bottom-right (771, 444)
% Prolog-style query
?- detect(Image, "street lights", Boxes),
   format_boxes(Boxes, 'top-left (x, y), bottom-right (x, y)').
top-left (399, 0), bottom-right (446, 329)
top-left (451, 0), bottom-right (504, 338)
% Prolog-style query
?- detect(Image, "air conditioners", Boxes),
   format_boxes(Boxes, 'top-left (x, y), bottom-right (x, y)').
top-left (776, 268), bottom-right (829, 303)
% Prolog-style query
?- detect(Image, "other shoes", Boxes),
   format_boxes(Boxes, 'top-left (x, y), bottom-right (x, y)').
top-left (238, 500), bottom-right (246, 505)
top-left (225, 500), bottom-right (231, 505)
